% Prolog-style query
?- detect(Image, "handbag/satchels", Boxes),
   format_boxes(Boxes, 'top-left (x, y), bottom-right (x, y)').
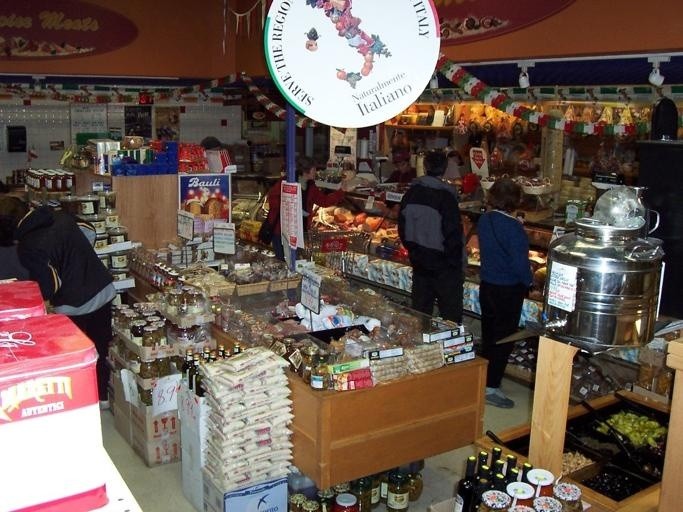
top-left (257, 207), bottom-right (280, 245)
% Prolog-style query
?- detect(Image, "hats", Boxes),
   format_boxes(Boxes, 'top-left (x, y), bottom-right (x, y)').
top-left (393, 151), bottom-right (410, 163)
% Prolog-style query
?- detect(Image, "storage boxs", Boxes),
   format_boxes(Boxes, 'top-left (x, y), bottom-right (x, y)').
top-left (113, 376), bottom-right (132, 446)
top-left (202, 467), bottom-right (288, 511)
top-left (129, 402), bottom-right (180, 467)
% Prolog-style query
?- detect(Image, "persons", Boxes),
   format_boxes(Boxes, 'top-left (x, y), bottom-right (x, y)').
top-left (0, 196), bottom-right (117, 411)
top-left (397, 149), bottom-right (468, 325)
top-left (432, 147), bottom-right (433, 149)
top-left (384, 152), bottom-right (417, 183)
top-left (267, 157), bottom-right (351, 262)
top-left (476, 178), bottom-right (533, 409)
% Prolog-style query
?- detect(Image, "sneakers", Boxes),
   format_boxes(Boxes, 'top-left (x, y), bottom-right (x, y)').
top-left (100, 398), bottom-right (110, 410)
top-left (484, 389), bottom-right (514, 409)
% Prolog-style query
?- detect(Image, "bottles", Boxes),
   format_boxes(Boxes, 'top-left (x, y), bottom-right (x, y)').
top-left (301, 350), bottom-right (317, 385)
top-left (217, 344), bottom-right (225, 357)
top-left (125, 312), bottom-right (140, 329)
top-left (290, 342), bottom-right (305, 372)
top-left (368, 473), bottom-right (380, 509)
top-left (151, 262), bottom-right (163, 287)
top-left (65, 172), bottom-right (76, 190)
top-left (156, 265), bottom-right (166, 290)
top-left (489, 459), bottom-right (505, 481)
top-left (166, 288), bottom-right (184, 317)
top-left (118, 308), bottom-right (134, 324)
top-left (489, 473), bottom-right (506, 492)
top-left (145, 315), bottom-right (160, 325)
top-left (310, 354), bottom-right (330, 390)
top-left (131, 319), bottom-right (148, 343)
top-left (469, 478), bottom-right (489, 512)
top-left (46, 173), bottom-right (56, 192)
top-left (477, 489), bottom-right (512, 512)
top-left (386, 471), bottom-right (411, 512)
top-left (520, 462), bottom-right (533, 484)
top-left (36, 173), bottom-right (44, 189)
top-left (489, 447), bottom-right (503, 472)
top-left (527, 468), bottom-right (555, 498)
top-left (505, 454), bottom-right (518, 478)
top-left (209, 352), bottom-right (217, 364)
top-left (332, 493), bottom-right (358, 512)
top-left (142, 325), bottom-right (161, 351)
top-left (479, 464), bottom-right (490, 477)
top-left (188, 354), bottom-right (200, 391)
top-left (301, 500), bottom-right (319, 512)
top-left (224, 349), bottom-right (232, 359)
top-left (317, 489), bottom-right (335, 512)
top-left (380, 469), bottom-right (393, 505)
top-left (140, 387), bottom-right (153, 406)
top-left (351, 477), bottom-right (372, 512)
top-left (283, 337), bottom-right (295, 362)
top-left (475, 451), bottom-right (488, 479)
top-left (56, 173), bottom-right (66, 191)
top-left (114, 304), bottom-right (129, 325)
top-left (155, 355), bottom-right (170, 377)
top-left (161, 267), bottom-right (171, 293)
top-left (139, 357), bottom-right (158, 378)
top-left (289, 494), bottom-right (307, 512)
top-left (553, 483), bottom-right (583, 512)
top-left (505, 467), bottom-right (520, 486)
top-left (181, 289), bottom-right (206, 315)
top-left (406, 471), bottom-right (424, 501)
top-left (221, 303), bottom-right (236, 332)
top-left (233, 341), bottom-right (241, 354)
top-left (506, 482), bottom-right (535, 508)
top-left (165, 271), bottom-right (180, 295)
top-left (453, 455), bottom-right (478, 512)
top-left (194, 359), bottom-right (207, 397)
top-left (214, 302), bottom-right (224, 330)
top-left (202, 346), bottom-right (210, 360)
top-left (533, 496), bottom-right (564, 512)
top-left (151, 320), bottom-right (168, 346)
top-left (169, 354), bottom-right (182, 375)
top-left (181, 349), bottom-right (194, 381)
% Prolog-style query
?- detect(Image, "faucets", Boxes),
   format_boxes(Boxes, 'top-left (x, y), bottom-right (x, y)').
top-left (541, 312), bottom-right (567, 336)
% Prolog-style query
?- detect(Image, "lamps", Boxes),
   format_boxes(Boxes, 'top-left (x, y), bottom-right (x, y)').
top-left (429, 75), bottom-right (437, 89)
top-left (517, 62), bottom-right (536, 88)
top-left (647, 57), bottom-right (670, 88)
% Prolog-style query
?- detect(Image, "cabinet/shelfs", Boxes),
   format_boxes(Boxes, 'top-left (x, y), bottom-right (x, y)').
top-left (474, 317), bottom-right (683, 512)
top-left (376, 100), bottom-right (682, 223)
top-left (126, 243), bottom-right (487, 488)
top-left (307, 180), bottom-right (638, 405)
top-left (73, 168), bottom-right (231, 246)
